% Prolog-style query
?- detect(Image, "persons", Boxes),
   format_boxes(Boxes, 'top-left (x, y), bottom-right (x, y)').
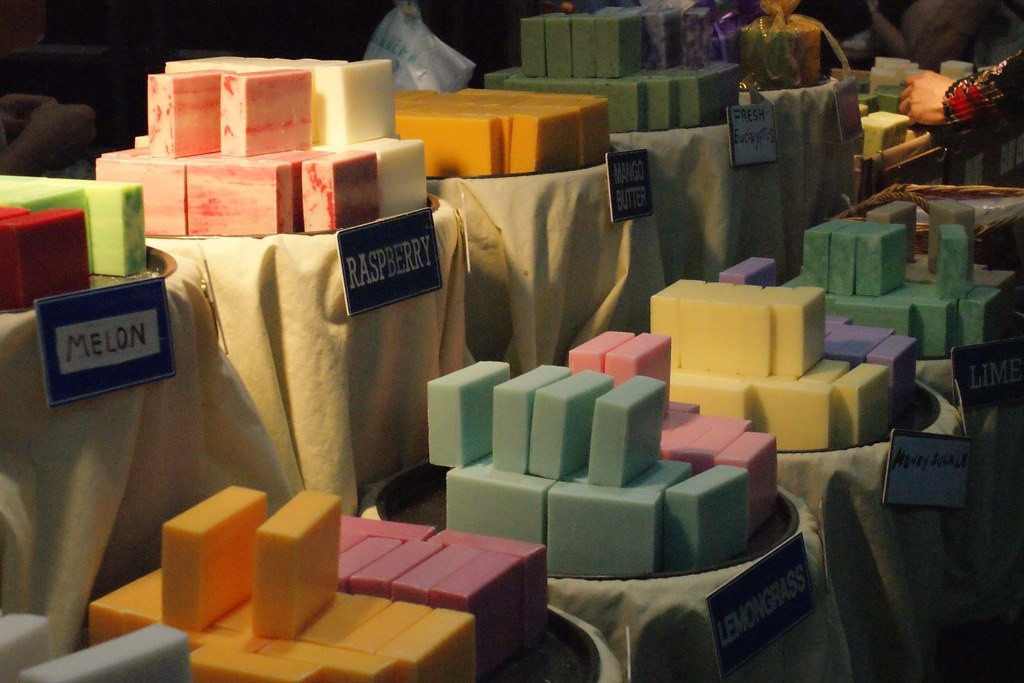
top-left (866, 0), bottom-right (1024, 73)
top-left (898, 48), bottom-right (1024, 126)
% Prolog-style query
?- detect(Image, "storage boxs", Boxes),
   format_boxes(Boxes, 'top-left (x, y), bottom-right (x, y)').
top-left (709, 0), bottom-right (821, 91)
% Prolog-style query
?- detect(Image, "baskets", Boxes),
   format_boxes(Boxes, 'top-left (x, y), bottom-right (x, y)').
top-left (833, 183), bottom-right (1024, 272)
